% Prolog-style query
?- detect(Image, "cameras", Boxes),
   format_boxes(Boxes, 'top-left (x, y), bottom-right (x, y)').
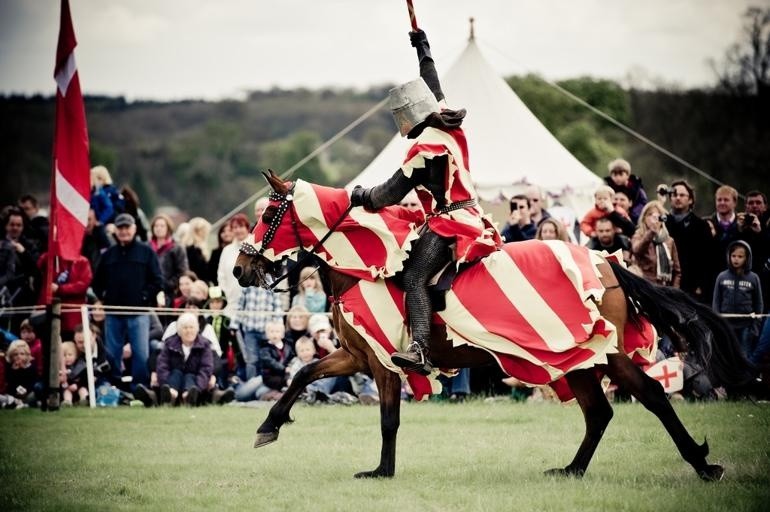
top-left (656, 216), bottom-right (667, 222)
top-left (743, 213), bottom-right (754, 225)
top-left (660, 188), bottom-right (677, 197)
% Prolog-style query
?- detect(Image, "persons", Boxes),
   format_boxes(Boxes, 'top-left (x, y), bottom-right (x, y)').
top-left (1, 166), bottom-right (495, 409)
top-left (495, 158), bottom-right (770, 406)
top-left (350, 28), bottom-right (505, 377)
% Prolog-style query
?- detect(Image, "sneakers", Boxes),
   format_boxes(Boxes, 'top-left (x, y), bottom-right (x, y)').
top-left (391, 350), bottom-right (431, 376)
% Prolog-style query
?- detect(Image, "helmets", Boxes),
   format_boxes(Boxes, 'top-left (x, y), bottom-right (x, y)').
top-left (389, 78), bottom-right (441, 137)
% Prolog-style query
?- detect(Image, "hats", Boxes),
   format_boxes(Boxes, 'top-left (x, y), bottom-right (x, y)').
top-left (113, 214), bottom-right (133, 227)
top-left (608, 159), bottom-right (630, 173)
top-left (309, 314), bottom-right (330, 334)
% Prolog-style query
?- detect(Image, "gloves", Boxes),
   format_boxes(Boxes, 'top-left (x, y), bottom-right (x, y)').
top-left (410, 28), bottom-right (433, 65)
top-left (350, 187), bottom-right (365, 207)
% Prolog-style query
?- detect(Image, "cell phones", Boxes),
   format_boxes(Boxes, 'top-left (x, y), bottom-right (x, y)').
top-left (511, 203), bottom-right (517, 214)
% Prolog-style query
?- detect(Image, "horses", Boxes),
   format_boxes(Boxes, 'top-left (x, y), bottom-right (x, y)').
top-left (233, 167), bottom-right (757, 483)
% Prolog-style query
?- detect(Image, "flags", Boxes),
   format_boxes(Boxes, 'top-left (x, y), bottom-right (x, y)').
top-left (46, 0), bottom-right (92, 262)
